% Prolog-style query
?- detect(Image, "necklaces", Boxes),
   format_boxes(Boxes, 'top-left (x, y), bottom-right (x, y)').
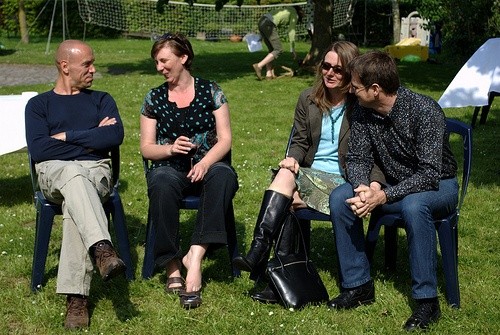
top-left (326, 103), bottom-right (345, 145)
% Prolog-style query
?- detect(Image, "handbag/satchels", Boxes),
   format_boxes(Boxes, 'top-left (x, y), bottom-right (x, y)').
top-left (266, 253), bottom-right (331, 311)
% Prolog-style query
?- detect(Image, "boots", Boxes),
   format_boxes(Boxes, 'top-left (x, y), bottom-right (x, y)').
top-left (233, 190), bottom-right (294, 276)
top-left (250, 211), bottom-right (302, 304)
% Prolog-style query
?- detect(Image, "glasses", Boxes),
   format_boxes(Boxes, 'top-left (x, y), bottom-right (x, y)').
top-left (161, 33), bottom-right (183, 44)
top-left (321, 61), bottom-right (345, 76)
top-left (349, 82), bottom-right (372, 93)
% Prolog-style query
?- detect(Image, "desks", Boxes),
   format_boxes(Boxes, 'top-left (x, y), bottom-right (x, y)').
top-left (437, 38), bottom-right (500, 127)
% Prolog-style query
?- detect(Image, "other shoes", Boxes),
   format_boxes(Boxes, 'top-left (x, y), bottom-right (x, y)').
top-left (94, 241), bottom-right (126, 281)
top-left (65, 294), bottom-right (90, 331)
top-left (267, 75), bottom-right (276, 79)
top-left (252, 63), bottom-right (262, 80)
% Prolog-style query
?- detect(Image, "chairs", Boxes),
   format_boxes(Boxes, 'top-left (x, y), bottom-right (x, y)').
top-left (27, 143), bottom-right (135, 294)
top-left (285, 123), bottom-right (331, 257)
top-left (366, 117), bottom-right (472, 310)
top-left (143, 149), bottom-right (241, 281)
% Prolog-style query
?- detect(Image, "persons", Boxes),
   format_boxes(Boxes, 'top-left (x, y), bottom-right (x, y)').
top-left (23, 39), bottom-right (127, 330)
top-left (231, 41), bottom-right (361, 302)
top-left (139, 31), bottom-right (239, 308)
top-left (328, 50), bottom-right (459, 332)
top-left (252, 5), bottom-right (305, 81)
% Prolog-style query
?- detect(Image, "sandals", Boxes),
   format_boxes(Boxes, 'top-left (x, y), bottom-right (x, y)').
top-left (179, 286), bottom-right (203, 309)
top-left (166, 277), bottom-right (185, 295)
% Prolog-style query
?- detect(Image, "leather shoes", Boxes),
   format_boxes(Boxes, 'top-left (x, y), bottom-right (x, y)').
top-left (328, 280), bottom-right (376, 311)
top-left (403, 303), bottom-right (442, 334)
